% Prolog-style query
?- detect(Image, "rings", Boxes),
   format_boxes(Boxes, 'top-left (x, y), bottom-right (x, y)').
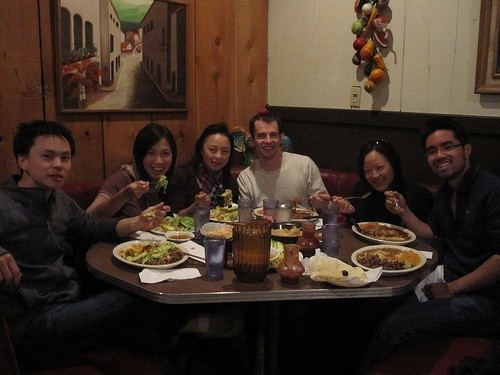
top-left (426, 290), bottom-right (428, 296)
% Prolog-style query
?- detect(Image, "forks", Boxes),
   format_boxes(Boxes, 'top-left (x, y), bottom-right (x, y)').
top-left (339, 192), bottom-right (372, 200)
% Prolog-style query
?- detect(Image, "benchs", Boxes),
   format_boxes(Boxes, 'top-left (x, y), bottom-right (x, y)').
top-left (0, 166), bottom-right (500, 375)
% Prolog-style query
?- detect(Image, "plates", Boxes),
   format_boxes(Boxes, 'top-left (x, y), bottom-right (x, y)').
top-left (209, 217), bottom-right (239, 223)
top-left (351, 244), bottom-right (427, 276)
top-left (253, 207), bottom-right (319, 222)
top-left (150, 228), bottom-right (195, 235)
top-left (352, 222), bottom-right (417, 246)
top-left (199, 221), bottom-right (235, 242)
top-left (112, 240), bottom-right (189, 270)
top-left (166, 231), bottom-right (195, 242)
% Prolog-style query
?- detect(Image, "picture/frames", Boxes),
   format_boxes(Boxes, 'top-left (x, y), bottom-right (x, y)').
top-left (49, 0), bottom-right (188, 116)
top-left (474, 1), bottom-right (500, 94)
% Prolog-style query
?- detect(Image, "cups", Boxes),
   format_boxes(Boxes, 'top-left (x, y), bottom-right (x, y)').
top-left (262, 199), bottom-right (279, 221)
top-left (322, 205), bottom-right (338, 224)
top-left (193, 206), bottom-right (210, 240)
top-left (322, 226), bottom-right (341, 258)
top-left (203, 236), bottom-right (226, 279)
top-left (238, 199), bottom-right (253, 222)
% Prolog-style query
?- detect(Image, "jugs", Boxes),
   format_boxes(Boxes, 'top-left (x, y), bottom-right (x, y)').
top-left (231, 220), bottom-right (272, 283)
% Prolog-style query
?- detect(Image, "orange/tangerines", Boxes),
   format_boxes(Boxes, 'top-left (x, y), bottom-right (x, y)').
top-left (370, 69), bottom-right (386, 84)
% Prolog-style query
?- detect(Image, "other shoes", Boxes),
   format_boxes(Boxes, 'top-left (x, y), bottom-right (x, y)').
top-left (195, 313), bottom-right (241, 338)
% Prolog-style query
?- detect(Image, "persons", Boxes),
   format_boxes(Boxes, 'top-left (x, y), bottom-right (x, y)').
top-left (179, 121), bottom-right (241, 217)
top-left (84, 123), bottom-right (185, 219)
top-left (354, 115), bottom-right (500, 375)
top-left (0, 119), bottom-right (250, 375)
top-left (236, 113), bottom-right (331, 223)
top-left (331, 139), bottom-right (437, 230)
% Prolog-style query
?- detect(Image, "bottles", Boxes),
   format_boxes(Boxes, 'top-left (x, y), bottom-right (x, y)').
top-left (276, 244), bottom-right (306, 284)
top-left (295, 223), bottom-right (321, 259)
top-left (277, 203), bottom-right (291, 222)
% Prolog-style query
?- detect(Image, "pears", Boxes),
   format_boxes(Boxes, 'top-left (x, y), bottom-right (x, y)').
top-left (351, 17), bottom-right (365, 34)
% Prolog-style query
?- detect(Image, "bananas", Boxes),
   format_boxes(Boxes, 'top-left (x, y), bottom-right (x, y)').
top-left (357, 6), bottom-right (378, 38)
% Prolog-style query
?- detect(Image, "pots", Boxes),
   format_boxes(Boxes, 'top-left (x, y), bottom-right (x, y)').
top-left (272, 224), bottom-right (302, 243)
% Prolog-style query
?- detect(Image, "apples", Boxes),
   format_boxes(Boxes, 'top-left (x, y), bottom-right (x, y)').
top-left (353, 38), bottom-right (368, 51)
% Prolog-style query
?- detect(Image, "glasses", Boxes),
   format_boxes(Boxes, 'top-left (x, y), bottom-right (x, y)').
top-left (425, 144), bottom-right (464, 159)
top-left (374, 140), bottom-right (385, 145)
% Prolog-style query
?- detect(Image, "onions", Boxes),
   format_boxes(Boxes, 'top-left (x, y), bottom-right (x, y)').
top-left (362, 3), bottom-right (372, 16)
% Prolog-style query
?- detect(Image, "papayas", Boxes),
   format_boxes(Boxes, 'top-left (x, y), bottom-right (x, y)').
top-left (360, 40), bottom-right (375, 60)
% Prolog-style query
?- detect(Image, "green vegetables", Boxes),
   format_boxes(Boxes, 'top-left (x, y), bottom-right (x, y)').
top-left (217, 190), bottom-right (239, 221)
top-left (156, 175), bottom-right (168, 194)
top-left (165, 215), bottom-right (195, 232)
top-left (124, 242), bottom-right (177, 265)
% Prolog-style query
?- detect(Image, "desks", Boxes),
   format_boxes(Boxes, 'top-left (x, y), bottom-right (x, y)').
top-left (85, 216), bottom-right (439, 375)
